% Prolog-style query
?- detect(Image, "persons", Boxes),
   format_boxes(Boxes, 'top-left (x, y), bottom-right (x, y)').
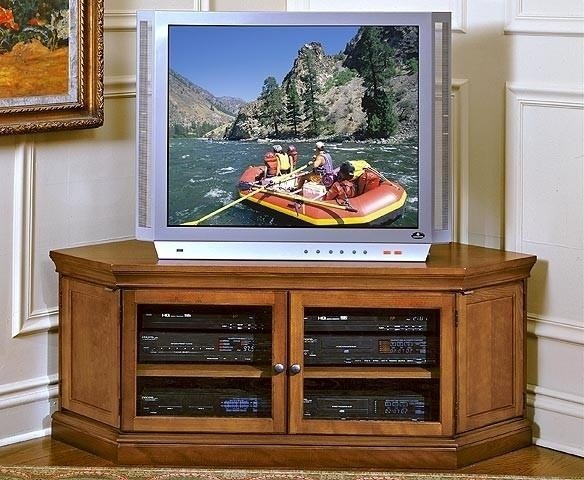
top-left (287, 145), bottom-right (298, 176)
top-left (292, 140), bottom-right (334, 191)
top-left (272, 144), bottom-right (291, 176)
top-left (256, 152), bottom-right (281, 182)
top-left (320, 161), bottom-right (358, 201)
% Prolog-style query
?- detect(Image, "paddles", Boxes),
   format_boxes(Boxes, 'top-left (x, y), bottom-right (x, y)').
top-left (237, 181), bottom-right (358, 213)
top-left (354, 159), bottom-right (400, 190)
top-left (178, 187), bottom-right (264, 225)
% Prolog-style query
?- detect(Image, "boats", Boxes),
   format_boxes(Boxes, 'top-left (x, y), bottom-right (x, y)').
top-left (237, 164), bottom-right (407, 230)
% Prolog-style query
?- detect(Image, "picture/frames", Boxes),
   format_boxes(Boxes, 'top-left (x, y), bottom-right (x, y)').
top-left (0, 0), bottom-right (106, 137)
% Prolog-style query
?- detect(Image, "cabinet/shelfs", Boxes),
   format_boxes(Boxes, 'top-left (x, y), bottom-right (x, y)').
top-left (44, 233), bottom-right (541, 468)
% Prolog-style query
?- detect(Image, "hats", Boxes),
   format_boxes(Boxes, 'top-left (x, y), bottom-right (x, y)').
top-left (315, 141), bottom-right (325, 151)
top-left (272, 144), bottom-right (283, 153)
top-left (340, 161), bottom-right (356, 177)
top-left (263, 152), bottom-right (276, 159)
top-left (286, 145), bottom-right (298, 155)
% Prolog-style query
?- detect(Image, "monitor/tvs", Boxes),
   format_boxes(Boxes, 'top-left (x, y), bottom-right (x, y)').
top-left (134, 10), bottom-right (452, 263)
